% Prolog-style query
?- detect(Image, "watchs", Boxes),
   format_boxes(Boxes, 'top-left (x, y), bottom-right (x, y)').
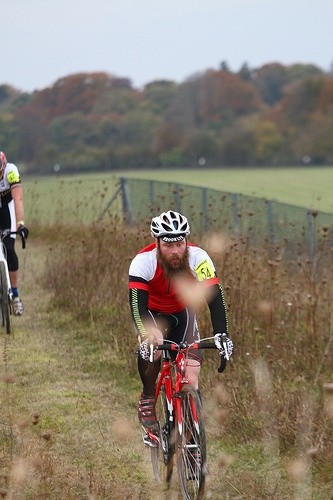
top-left (17, 221), bottom-right (24, 226)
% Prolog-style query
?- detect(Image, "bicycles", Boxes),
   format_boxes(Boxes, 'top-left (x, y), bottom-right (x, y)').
top-left (0, 227), bottom-right (26, 336)
top-left (140, 332), bottom-right (234, 500)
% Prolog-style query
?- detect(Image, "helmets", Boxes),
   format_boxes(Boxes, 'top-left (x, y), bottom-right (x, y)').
top-left (0, 151), bottom-right (7, 175)
top-left (151, 211), bottom-right (191, 242)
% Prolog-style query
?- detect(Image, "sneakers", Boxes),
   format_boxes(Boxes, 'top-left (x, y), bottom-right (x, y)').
top-left (12, 297), bottom-right (24, 316)
top-left (187, 444), bottom-right (201, 473)
top-left (138, 393), bottom-right (157, 426)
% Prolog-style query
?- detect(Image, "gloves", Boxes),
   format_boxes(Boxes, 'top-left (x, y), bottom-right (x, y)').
top-left (140, 339), bottom-right (158, 360)
top-left (16, 221), bottom-right (28, 239)
top-left (214, 333), bottom-right (233, 358)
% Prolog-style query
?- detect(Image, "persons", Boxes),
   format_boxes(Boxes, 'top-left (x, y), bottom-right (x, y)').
top-left (129, 210), bottom-right (234, 477)
top-left (0, 151), bottom-right (25, 316)
